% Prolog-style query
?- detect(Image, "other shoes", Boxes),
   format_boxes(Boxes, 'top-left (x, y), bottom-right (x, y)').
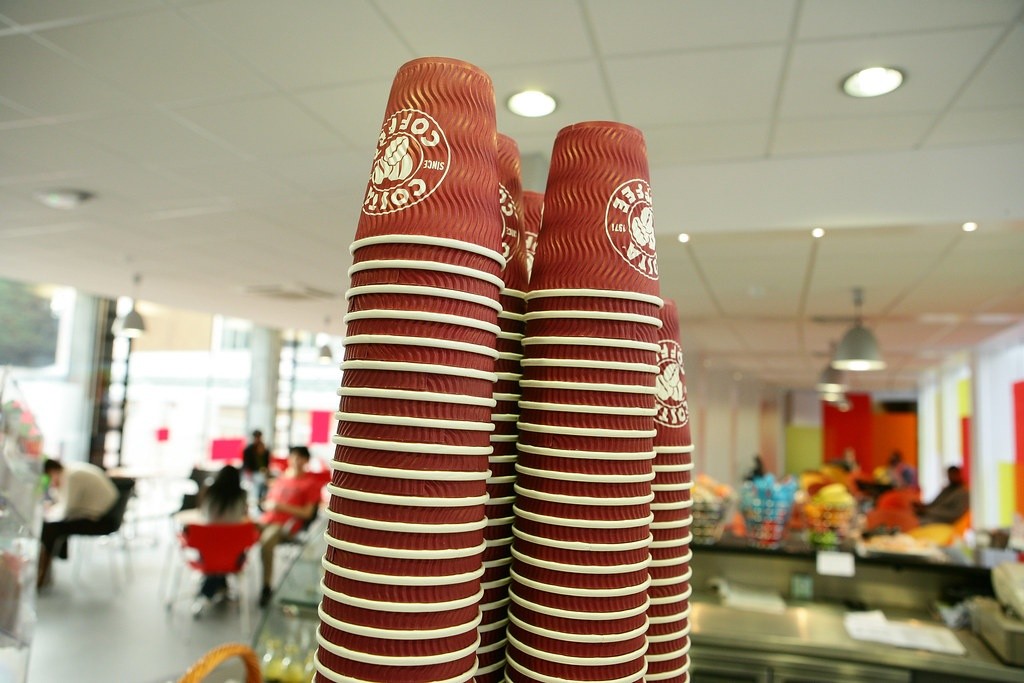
top-left (254, 586), bottom-right (269, 608)
top-left (226, 578), bottom-right (238, 602)
top-left (192, 592), bottom-right (222, 616)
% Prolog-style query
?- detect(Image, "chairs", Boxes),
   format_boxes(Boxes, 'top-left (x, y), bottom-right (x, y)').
top-left (269, 504), bottom-right (321, 596)
top-left (158, 467), bottom-right (216, 515)
top-left (158, 522), bottom-right (264, 615)
top-left (70, 475), bottom-right (137, 591)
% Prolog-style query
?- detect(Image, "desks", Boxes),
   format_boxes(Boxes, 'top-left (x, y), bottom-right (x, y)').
top-left (690, 544), bottom-right (995, 612)
top-left (691, 603), bottom-right (1024, 683)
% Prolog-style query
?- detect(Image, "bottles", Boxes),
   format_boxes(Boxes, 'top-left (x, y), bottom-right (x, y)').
top-left (249, 486), bottom-right (332, 683)
top-left (0, 365), bottom-right (45, 683)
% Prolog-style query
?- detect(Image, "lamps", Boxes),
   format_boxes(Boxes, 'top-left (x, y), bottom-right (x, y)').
top-left (830, 287), bottom-right (888, 373)
top-left (817, 343), bottom-right (849, 405)
top-left (506, 89), bottom-right (560, 120)
top-left (839, 65), bottom-right (907, 99)
top-left (31, 188), bottom-right (90, 212)
top-left (119, 298), bottom-right (146, 331)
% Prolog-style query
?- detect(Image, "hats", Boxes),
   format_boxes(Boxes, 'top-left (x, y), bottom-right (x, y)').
top-left (289, 447), bottom-right (310, 458)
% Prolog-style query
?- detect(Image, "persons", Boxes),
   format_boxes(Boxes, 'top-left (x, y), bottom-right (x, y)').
top-left (872, 451), bottom-right (921, 532)
top-left (742, 454), bottom-right (764, 482)
top-left (178, 430), bottom-right (321, 608)
top-left (912, 466), bottom-right (969, 525)
top-left (37, 459), bottom-right (121, 593)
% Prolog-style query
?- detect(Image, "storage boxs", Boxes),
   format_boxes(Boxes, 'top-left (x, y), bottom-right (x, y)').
top-left (972, 597), bottom-right (1024, 668)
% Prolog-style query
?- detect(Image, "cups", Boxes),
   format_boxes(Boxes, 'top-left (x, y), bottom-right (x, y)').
top-left (311, 56), bottom-right (694, 683)
